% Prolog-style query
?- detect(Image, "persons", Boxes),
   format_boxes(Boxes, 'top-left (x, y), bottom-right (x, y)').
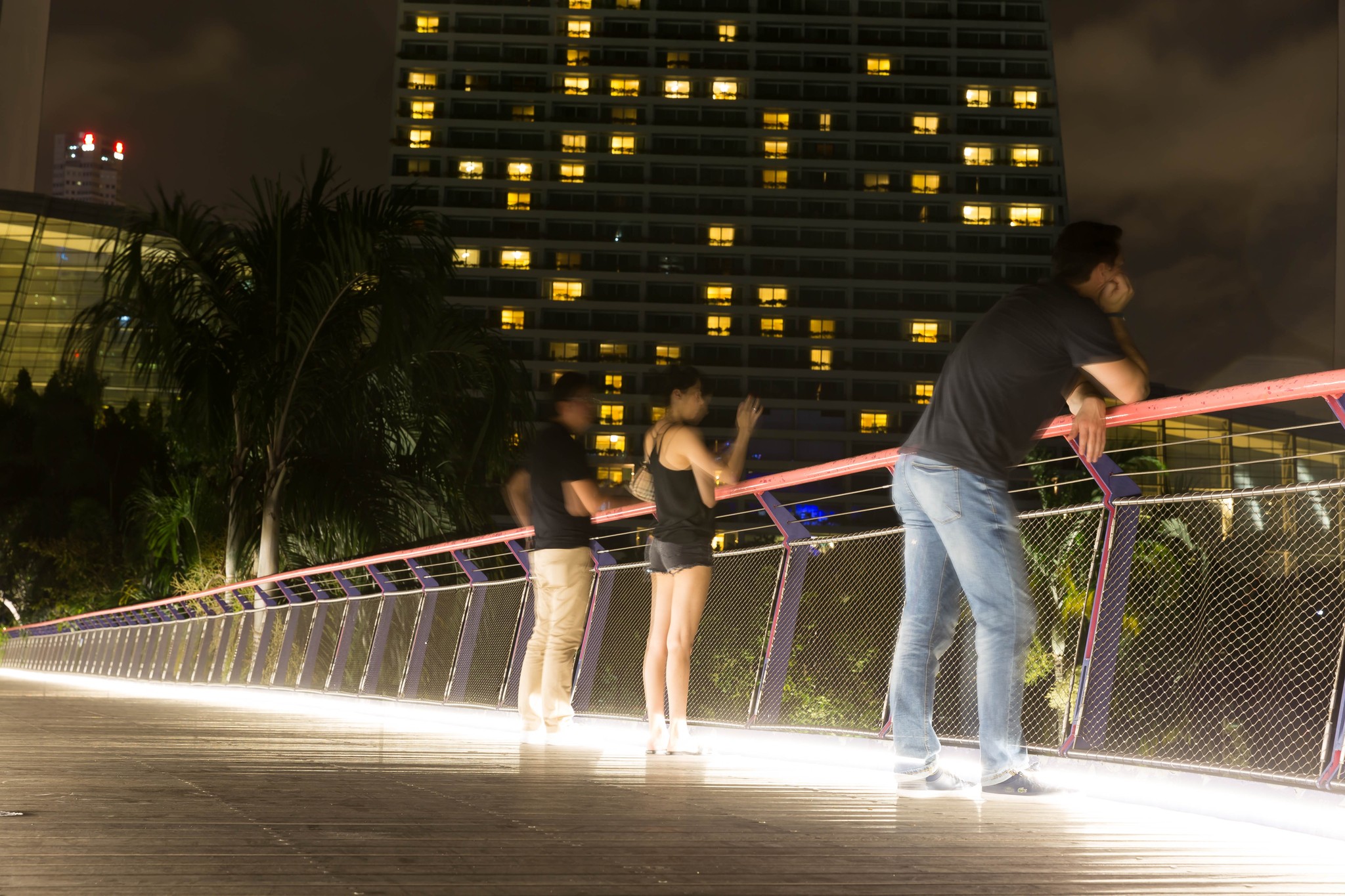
top-left (891, 223), bottom-right (1150, 798)
top-left (644, 364), bottom-right (763, 754)
top-left (508, 372), bottom-right (642, 746)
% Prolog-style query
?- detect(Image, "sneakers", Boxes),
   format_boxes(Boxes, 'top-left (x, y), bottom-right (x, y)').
top-left (897, 767), bottom-right (976, 798)
top-left (982, 772), bottom-right (1059, 805)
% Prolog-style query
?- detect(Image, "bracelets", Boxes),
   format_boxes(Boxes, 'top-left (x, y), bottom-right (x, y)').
top-left (1106, 311), bottom-right (1124, 318)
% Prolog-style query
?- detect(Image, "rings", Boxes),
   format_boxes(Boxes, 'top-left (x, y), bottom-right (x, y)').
top-left (752, 407), bottom-right (757, 411)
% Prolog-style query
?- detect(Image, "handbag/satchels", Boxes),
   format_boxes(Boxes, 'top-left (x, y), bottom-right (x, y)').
top-left (629, 461), bottom-right (656, 503)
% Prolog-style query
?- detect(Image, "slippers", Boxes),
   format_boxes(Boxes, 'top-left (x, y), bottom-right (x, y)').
top-left (666, 745), bottom-right (702, 755)
top-left (646, 750), bottom-right (664, 756)
